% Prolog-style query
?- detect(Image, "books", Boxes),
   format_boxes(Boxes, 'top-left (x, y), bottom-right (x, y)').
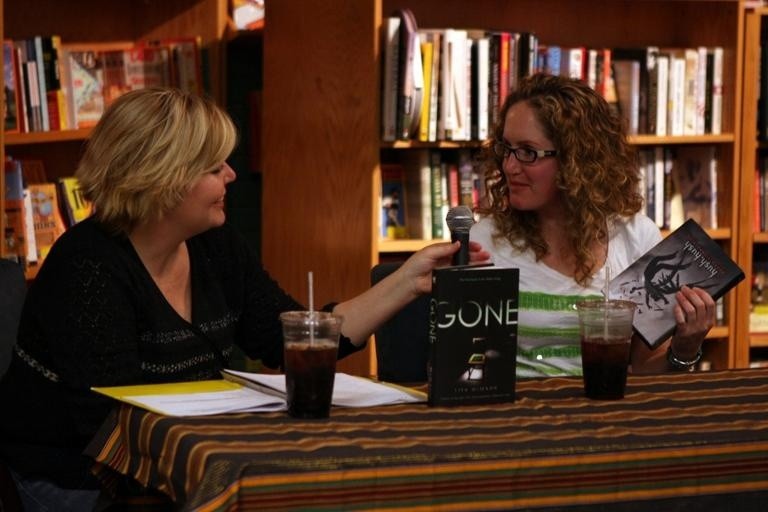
top-left (382, 147), bottom-right (501, 240)
top-left (3, 154), bottom-right (95, 280)
top-left (600, 218), bottom-right (745, 352)
top-left (716, 296), bottom-right (723, 326)
top-left (750, 258), bottom-right (768, 336)
top-left (637, 146), bottom-right (718, 233)
top-left (431, 263), bottom-right (520, 405)
top-left (0, 33), bottom-right (211, 132)
top-left (755, 168), bottom-right (768, 234)
top-left (384, 8), bottom-right (726, 138)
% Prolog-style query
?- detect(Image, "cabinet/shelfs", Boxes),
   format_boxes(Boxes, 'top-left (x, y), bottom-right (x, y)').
top-left (0, 0), bottom-right (228, 274)
top-left (263, 0), bottom-right (767, 379)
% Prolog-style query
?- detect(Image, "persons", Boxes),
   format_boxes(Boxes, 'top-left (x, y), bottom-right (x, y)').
top-left (465, 72), bottom-right (718, 376)
top-left (2, 88), bottom-right (490, 512)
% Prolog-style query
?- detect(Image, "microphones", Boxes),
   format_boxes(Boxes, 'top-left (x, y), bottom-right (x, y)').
top-left (446, 206), bottom-right (475, 265)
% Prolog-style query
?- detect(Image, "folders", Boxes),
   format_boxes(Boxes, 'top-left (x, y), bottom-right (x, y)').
top-left (92, 368), bottom-right (428, 417)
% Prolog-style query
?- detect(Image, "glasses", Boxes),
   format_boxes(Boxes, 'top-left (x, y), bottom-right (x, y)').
top-left (494, 142), bottom-right (558, 163)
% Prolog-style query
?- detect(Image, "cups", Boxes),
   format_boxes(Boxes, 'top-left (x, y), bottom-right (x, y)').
top-left (279, 311), bottom-right (343, 421)
top-left (575, 300), bottom-right (638, 401)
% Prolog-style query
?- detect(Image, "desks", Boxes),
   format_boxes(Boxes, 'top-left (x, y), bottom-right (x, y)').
top-left (85, 366), bottom-right (768, 511)
